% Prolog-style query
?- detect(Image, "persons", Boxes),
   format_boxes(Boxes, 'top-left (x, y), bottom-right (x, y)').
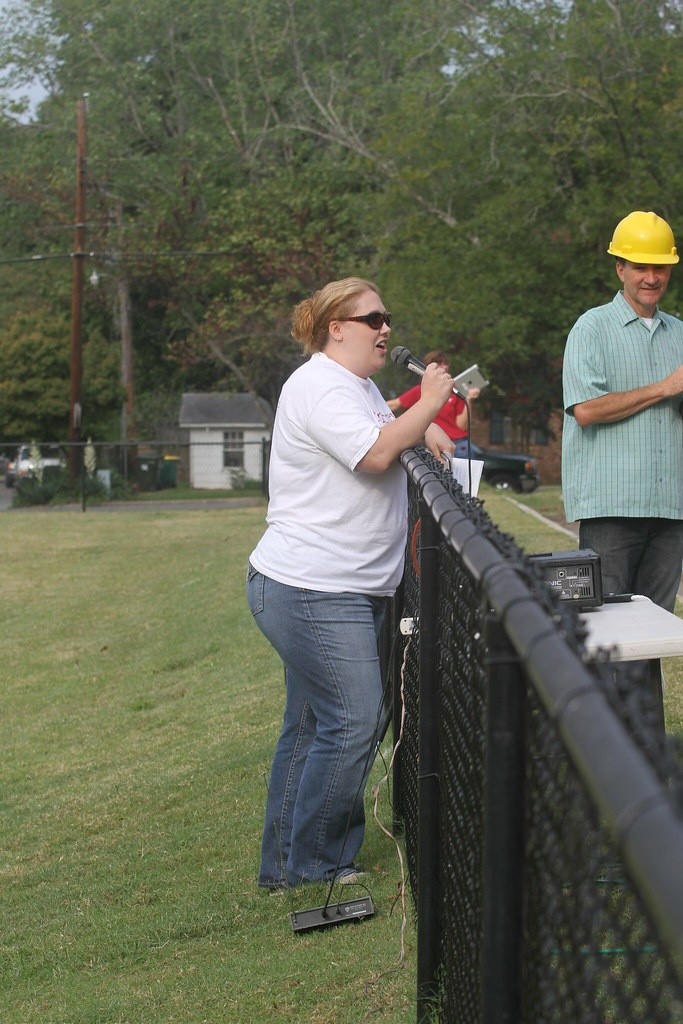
top-left (386, 350), bottom-right (480, 461)
top-left (558, 210), bottom-right (682, 610)
top-left (245, 278), bottom-right (458, 896)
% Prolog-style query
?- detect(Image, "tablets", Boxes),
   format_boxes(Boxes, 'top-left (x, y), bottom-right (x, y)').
top-left (452, 363), bottom-right (490, 398)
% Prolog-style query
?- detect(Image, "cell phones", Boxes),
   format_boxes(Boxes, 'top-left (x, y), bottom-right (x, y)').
top-left (440, 451), bottom-right (451, 472)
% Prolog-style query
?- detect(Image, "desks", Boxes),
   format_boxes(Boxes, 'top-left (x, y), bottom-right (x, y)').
top-left (580, 592), bottom-right (683, 747)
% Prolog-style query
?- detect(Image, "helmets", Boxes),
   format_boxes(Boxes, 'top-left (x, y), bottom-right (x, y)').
top-left (607, 211), bottom-right (680, 264)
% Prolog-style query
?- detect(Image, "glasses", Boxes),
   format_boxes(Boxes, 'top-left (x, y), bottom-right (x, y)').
top-left (331, 311), bottom-right (393, 331)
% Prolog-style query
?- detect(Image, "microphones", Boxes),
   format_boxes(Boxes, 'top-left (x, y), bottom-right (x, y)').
top-left (390, 346), bottom-right (466, 400)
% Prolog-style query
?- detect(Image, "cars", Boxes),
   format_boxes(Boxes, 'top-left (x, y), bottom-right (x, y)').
top-left (453, 439), bottom-right (541, 494)
top-left (6, 444), bottom-right (67, 489)
